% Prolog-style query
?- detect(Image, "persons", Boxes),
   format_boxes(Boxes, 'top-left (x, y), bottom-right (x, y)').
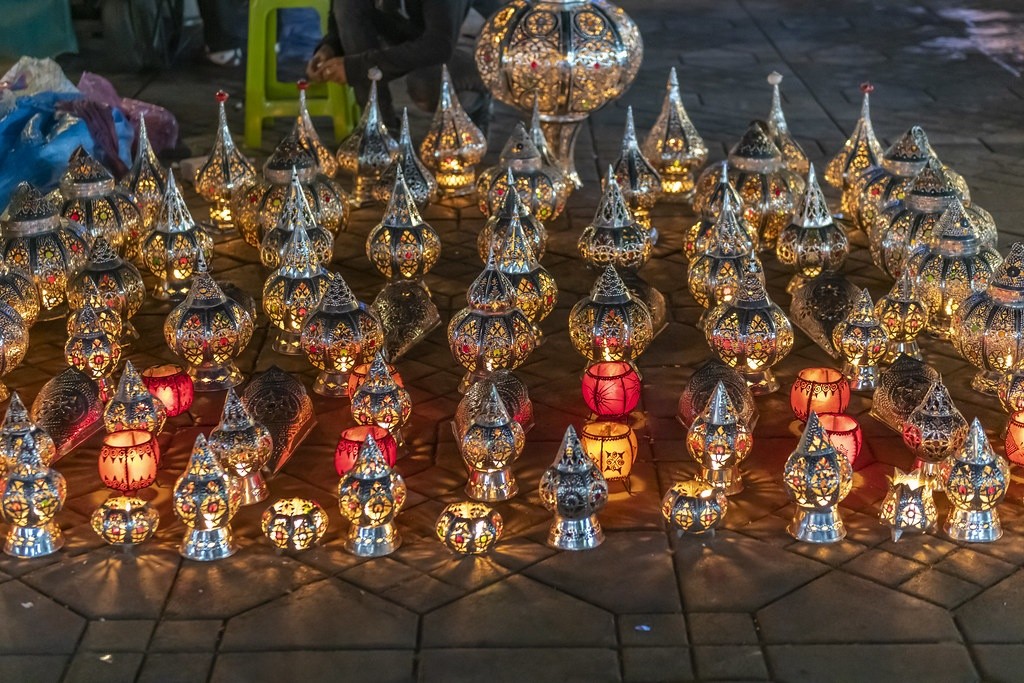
top-left (183, 0), bottom-right (286, 81)
top-left (304, 0), bottom-right (494, 145)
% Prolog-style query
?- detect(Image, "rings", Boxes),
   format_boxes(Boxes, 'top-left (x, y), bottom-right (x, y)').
top-left (326, 68), bottom-right (330, 75)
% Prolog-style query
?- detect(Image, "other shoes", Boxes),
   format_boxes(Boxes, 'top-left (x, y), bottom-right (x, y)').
top-left (187, 43), bottom-right (243, 67)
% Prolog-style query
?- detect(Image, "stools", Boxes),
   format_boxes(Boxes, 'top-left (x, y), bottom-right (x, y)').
top-left (244, 0), bottom-right (363, 150)
